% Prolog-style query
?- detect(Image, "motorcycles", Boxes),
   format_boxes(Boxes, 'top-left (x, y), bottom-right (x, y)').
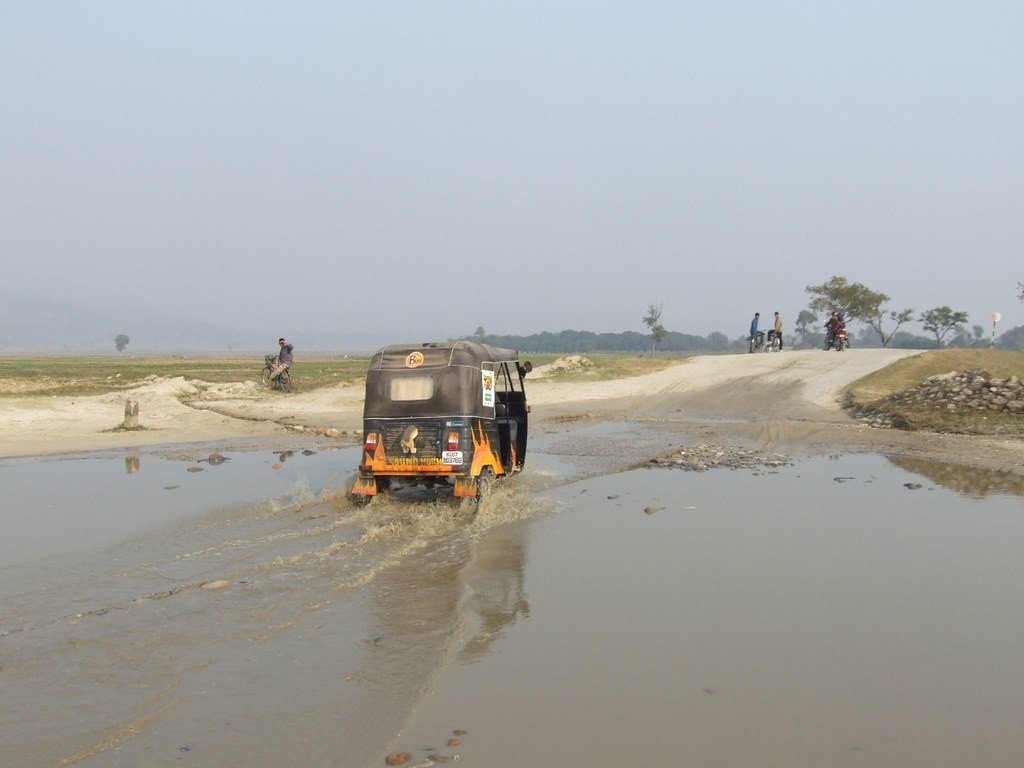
top-left (352, 341), bottom-right (532, 512)
top-left (825, 319), bottom-right (848, 352)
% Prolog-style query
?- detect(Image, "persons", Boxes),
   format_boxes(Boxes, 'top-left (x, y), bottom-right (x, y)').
top-left (767, 312), bottom-right (783, 352)
top-left (269, 338), bottom-right (294, 380)
top-left (495, 402), bottom-right (522, 472)
top-left (750, 313), bottom-right (765, 352)
top-left (823, 311), bottom-right (846, 351)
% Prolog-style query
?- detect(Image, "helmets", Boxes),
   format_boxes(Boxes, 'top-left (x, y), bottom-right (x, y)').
top-left (832, 312), bottom-right (838, 316)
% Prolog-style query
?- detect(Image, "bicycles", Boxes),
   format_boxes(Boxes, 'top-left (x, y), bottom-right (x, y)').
top-left (748, 333), bottom-right (765, 353)
top-left (261, 353), bottom-right (293, 393)
top-left (763, 332), bottom-right (781, 353)
